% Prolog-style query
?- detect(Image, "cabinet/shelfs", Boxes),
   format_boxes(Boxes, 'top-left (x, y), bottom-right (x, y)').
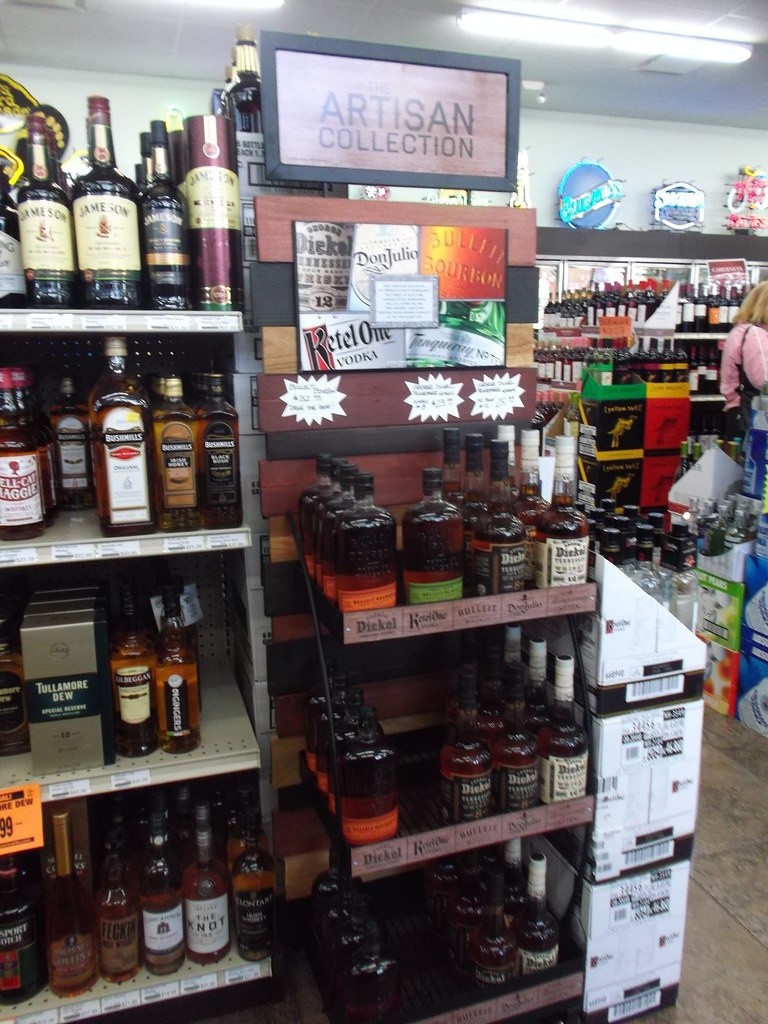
top-left (291, 566), bottom-right (586, 1022)
top-left (1, 304), bottom-right (284, 1021)
top-left (535, 324), bottom-right (732, 401)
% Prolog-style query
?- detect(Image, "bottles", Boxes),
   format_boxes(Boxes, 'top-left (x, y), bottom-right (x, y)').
top-left (0, 28), bottom-right (591, 1024)
top-left (538, 268), bottom-right (759, 578)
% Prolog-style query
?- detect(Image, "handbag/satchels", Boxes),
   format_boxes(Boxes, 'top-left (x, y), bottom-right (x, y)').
top-left (735, 325), bottom-right (761, 429)
top-left (667, 435), bottom-right (745, 512)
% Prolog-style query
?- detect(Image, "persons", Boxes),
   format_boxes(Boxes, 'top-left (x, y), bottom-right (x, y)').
top-left (720, 280), bottom-right (768, 439)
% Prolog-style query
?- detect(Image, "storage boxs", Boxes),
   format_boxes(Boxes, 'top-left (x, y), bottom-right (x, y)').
top-left (564, 397), bottom-right (767, 1022)
